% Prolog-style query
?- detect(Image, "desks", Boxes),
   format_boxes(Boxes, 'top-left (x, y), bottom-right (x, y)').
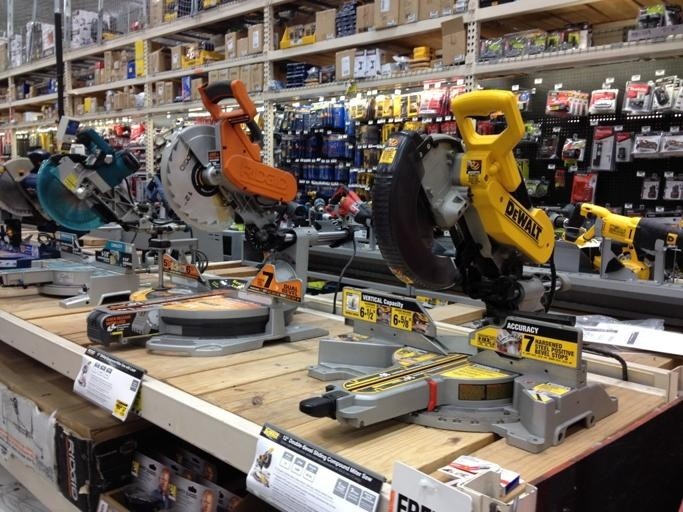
top-left (2, 291), bottom-right (682, 512)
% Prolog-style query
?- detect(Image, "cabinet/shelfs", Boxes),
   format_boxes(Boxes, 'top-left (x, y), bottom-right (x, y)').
top-left (0, 1), bottom-right (681, 130)
